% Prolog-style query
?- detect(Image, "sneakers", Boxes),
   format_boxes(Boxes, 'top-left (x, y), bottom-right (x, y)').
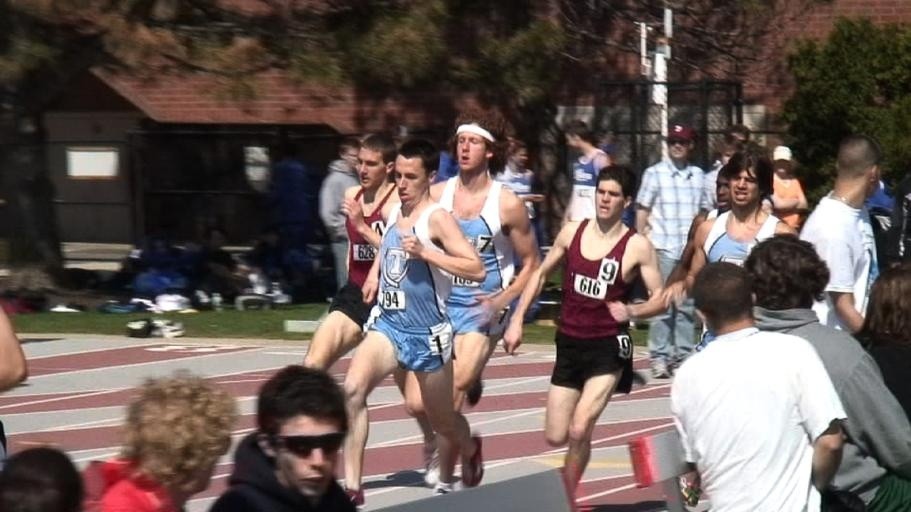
top-left (650, 358), bottom-right (670, 378)
top-left (424, 438), bottom-right (482, 497)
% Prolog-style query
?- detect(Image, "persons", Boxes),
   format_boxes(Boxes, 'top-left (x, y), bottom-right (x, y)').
top-left (260, 226), bottom-right (285, 278)
top-left (303, 116), bottom-right (911, 511)
top-left (84, 373), bottom-right (236, 512)
top-left (209, 364), bottom-right (358, 510)
top-left (0, 303), bottom-right (27, 459)
top-left (185, 228), bottom-right (233, 309)
top-left (135, 238), bottom-right (184, 293)
top-left (1, 449), bottom-right (83, 512)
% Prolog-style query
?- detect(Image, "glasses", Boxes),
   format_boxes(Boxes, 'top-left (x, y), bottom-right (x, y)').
top-left (273, 433), bottom-right (346, 455)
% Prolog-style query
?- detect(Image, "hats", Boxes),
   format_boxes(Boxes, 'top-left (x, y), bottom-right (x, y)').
top-left (668, 124), bottom-right (697, 141)
top-left (773, 146), bottom-right (791, 161)
